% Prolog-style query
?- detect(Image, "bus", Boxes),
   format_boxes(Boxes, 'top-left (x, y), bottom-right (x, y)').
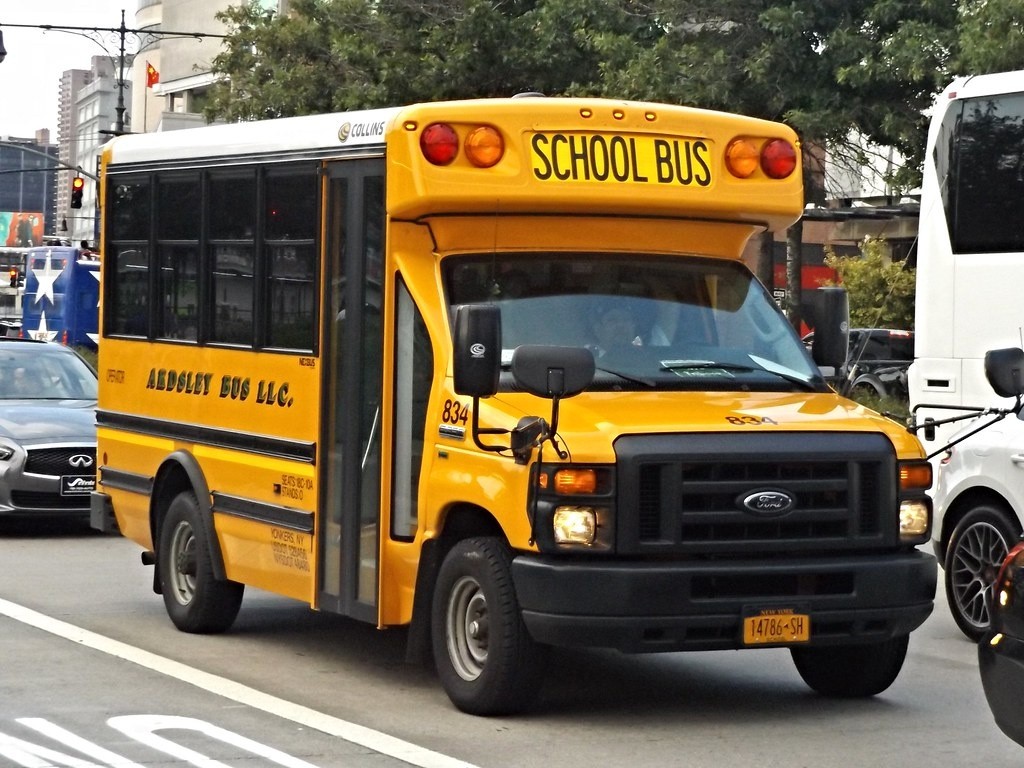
top-left (914, 69), bottom-right (1022, 521)
top-left (91, 94), bottom-right (1023, 716)
top-left (17, 244), bottom-right (102, 351)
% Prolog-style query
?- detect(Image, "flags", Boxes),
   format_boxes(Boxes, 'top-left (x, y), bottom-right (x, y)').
top-left (147, 63), bottom-right (159, 88)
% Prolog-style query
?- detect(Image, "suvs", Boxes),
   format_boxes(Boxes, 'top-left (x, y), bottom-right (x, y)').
top-left (799, 327), bottom-right (919, 406)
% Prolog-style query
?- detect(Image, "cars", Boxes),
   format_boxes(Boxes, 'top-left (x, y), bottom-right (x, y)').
top-left (975, 544), bottom-right (1024, 742)
top-left (928, 397), bottom-right (1024, 641)
top-left (0, 337), bottom-right (104, 512)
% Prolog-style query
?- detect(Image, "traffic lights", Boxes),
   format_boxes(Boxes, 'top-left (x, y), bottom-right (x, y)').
top-left (10, 270), bottom-right (24, 287)
top-left (71, 176), bottom-right (84, 209)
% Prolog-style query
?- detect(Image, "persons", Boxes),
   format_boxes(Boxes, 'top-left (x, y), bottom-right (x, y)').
top-left (24, 213), bottom-right (35, 247)
top-left (580, 267), bottom-right (682, 359)
top-left (12, 366), bottom-right (40, 396)
top-left (78, 240), bottom-right (92, 253)
top-left (13, 212), bottom-right (25, 247)
